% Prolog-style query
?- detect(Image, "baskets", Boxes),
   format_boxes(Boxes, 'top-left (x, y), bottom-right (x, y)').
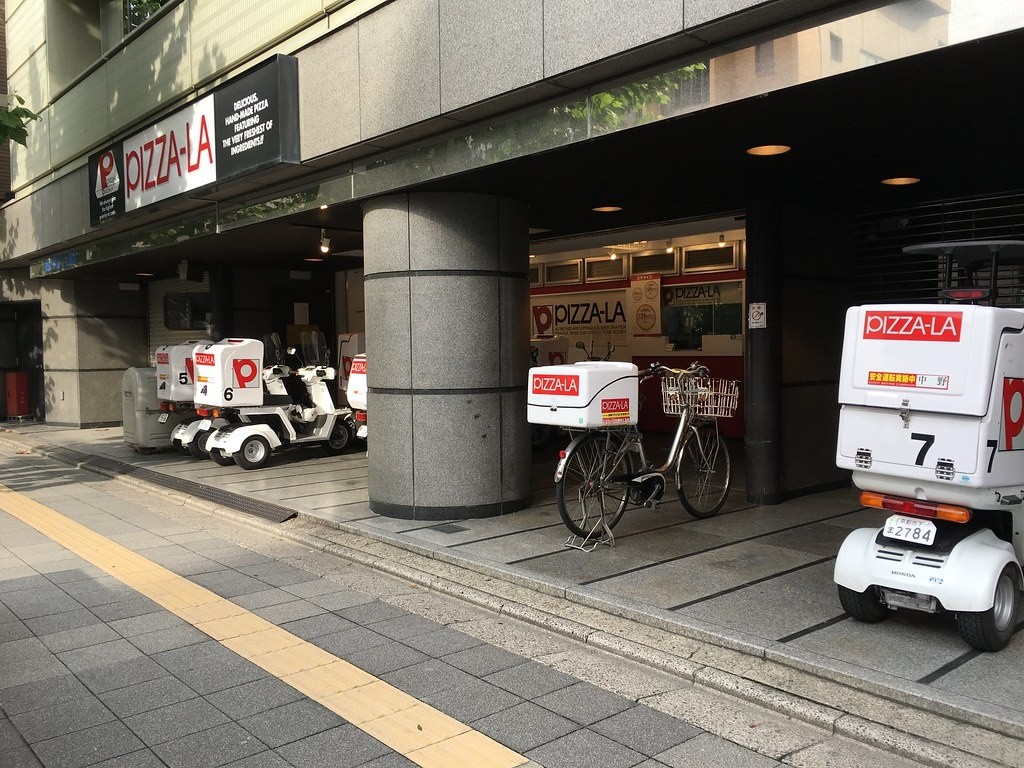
top-left (662, 376), bottom-right (738, 417)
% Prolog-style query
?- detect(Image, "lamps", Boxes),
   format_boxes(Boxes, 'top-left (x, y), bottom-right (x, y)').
top-left (744, 108), bottom-right (791, 157)
top-left (320, 230), bottom-right (330, 253)
top-left (879, 159), bottom-right (920, 185)
top-left (607, 248), bottom-right (617, 261)
top-left (718, 235), bottom-right (726, 248)
top-left (666, 238), bottom-right (674, 253)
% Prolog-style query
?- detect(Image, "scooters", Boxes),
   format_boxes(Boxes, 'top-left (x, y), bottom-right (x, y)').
top-left (833, 240), bottom-right (1024, 652)
top-left (156, 334), bottom-right (369, 471)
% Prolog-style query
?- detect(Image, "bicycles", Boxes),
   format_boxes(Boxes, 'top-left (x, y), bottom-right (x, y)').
top-left (526, 358), bottom-right (741, 553)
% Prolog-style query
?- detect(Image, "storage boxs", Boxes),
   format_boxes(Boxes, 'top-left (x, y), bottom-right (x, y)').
top-left (529, 333), bottom-right (571, 366)
top-left (155, 340), bottom-right (215, 402)
top-left (527, 361), bottom-right (638, 429)
top-left (835, 301), bottom-right (1024, 488)
top-left (337, 332), bottom-right (365, 390)
top-left (193, 338), bottom-right (264, 407)
top-left (347, 353), bottom-right (367, 410)
